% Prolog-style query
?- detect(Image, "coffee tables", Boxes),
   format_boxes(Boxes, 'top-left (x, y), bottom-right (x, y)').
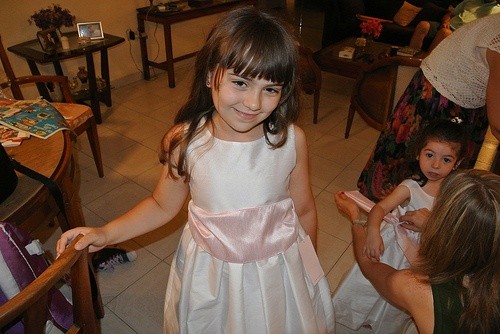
top-left (311, 38), bottom-right (425, 124)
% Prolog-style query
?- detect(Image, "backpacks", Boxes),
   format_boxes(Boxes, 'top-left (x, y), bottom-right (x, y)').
top-left (0, 223), bottom-right (74, 334)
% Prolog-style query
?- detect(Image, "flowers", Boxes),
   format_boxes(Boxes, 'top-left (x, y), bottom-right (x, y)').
top-left (357, 19), bottom-right (382, 45)
top-left (27, 4), bottom-right (76, 28)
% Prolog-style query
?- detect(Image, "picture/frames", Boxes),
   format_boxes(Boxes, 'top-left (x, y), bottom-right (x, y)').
top-left (76, 22), bottom-right (104, 41)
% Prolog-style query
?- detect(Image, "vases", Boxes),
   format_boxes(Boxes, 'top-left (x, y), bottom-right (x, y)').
top-left (360, 36), bottom-right (373, 59)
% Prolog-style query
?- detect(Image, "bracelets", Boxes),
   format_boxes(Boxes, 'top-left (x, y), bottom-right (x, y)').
top-left (350, 219), bottom-right (366, 227)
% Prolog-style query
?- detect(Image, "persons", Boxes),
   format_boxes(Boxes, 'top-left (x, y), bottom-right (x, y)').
top-left (54, 17), bottom-right (336, 334)
top-left (409, 0), bottom-right (500, 51)
top-left (361, 113), bottom-right (468, 334)
top-left (357, 12), bottom-right (500, 205)
top-left (334, 168), bottom-right (500, 334)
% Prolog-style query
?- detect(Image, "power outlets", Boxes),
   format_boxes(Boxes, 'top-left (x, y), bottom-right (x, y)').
top-left (126, 31), bottom-right (139, 39)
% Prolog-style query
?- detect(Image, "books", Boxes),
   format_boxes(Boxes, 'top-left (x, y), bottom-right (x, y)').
top-left (397, 47), bottom-right (418, 57)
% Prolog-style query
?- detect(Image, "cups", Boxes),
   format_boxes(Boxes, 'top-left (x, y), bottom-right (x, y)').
top-left (390, 46), bottom-right (400, 56)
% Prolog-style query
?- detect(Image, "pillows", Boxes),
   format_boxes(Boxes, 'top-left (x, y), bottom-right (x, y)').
top-left (393, 0), bottom-right (423, 27)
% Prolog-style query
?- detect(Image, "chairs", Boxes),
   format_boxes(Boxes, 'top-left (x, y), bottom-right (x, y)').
top-left (0, 75), bottom-right (105, 180)
top-left (344, 56), bottom-right (424, 141)
top-left (0, 225), bottom-right (100, 334)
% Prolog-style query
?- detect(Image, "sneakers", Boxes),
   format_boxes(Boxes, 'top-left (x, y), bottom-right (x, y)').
top-left (94, 247), bottom-right (138, 272)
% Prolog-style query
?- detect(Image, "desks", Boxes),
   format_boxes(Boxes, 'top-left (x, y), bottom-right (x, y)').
top-left (0, 98), bottom-right (105, 320)
top-left (7, 32), bottom-right (126, 125)
top-left (136, 1), bottom-right (257, 89)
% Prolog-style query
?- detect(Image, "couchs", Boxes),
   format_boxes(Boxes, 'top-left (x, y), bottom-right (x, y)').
top-left (323, 0), bottom-right (416, 47)
